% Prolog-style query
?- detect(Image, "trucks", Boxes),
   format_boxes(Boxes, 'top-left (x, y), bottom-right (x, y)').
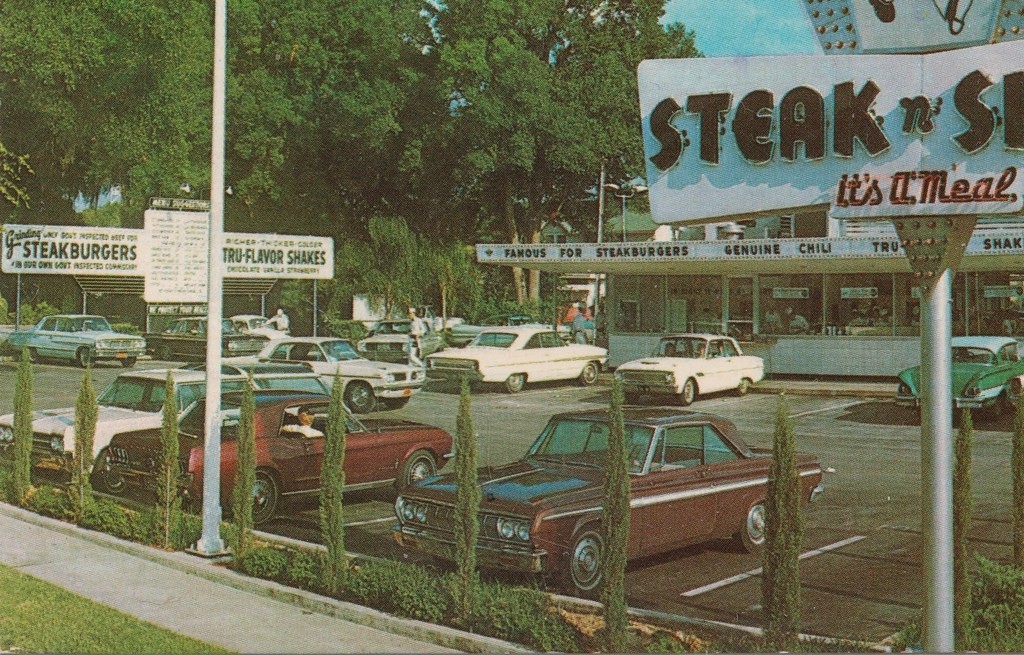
top-left (556, 272), bottom-right (606, 312)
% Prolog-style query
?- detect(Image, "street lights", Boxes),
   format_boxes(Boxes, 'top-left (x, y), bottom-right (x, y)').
top-left (603, 183), bottom-right (649, 241)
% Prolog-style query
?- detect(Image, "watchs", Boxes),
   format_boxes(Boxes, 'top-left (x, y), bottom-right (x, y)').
top-left (260, 308), bottom-right (290, 334)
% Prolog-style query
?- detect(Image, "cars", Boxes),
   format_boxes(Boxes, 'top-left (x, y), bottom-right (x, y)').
top-left (229, 315), bottom-right (289, 340)
top-left (101, 387), bottom-right (457, 530)
top-left (389, 405), bottom-right (826, 602)
top-left (355, 318), bottom-right (444, 359)
top-left (142, 315), bottom-right (271, 361)
top-left (9, 313), bottom-right (146, 369)
top-left (893, 336), bottom-right (1024, 419)
top-left (221, 337), bottom-right (425, 414)
top-left (613, 332), bottom-right (765, 406)
top-left (0, 359), bottom-right (351, 496)
top-left (446, 313), bottom-right (575, 349)
top-left (423, 325), bottom-right (611, 394)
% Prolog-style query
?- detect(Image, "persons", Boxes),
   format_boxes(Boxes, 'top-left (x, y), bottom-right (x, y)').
top-left (571, 302), bottom-right (607, 345)
top-left (279, 406), bottom-right (325, 438)
top-left (761, 303), bottom-right (783, 334)
top-left (190, 325), bottom-right (201, 334)
top-left (409, 310), bottom-right (427, 362)
top-left (236, 321), bottom-right (260, 335)
top-left (852, 304), bottom-right (923, 327)
top-left (785, 306), bottom-right (809, 335)
top-left (663, 340), bottom-right (729, 356)
